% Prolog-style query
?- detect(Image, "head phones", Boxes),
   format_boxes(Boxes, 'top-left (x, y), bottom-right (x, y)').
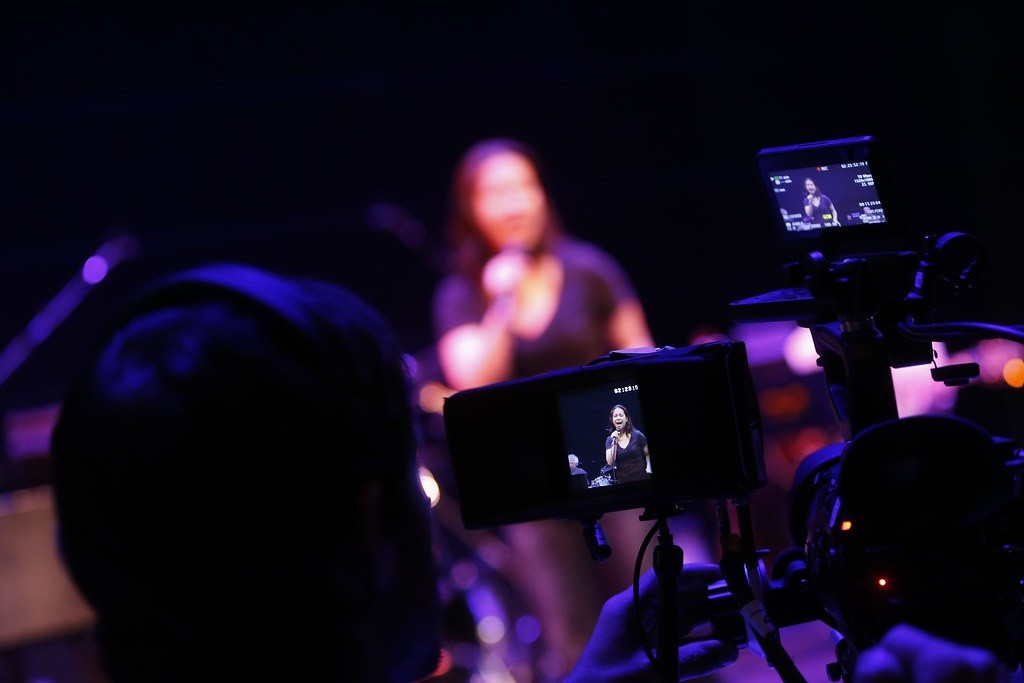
top-left (82, 262), bottom-right (437, 658)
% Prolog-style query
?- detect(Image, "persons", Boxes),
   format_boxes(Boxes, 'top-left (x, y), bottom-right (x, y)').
top-left (567, 454), bottom-right (589, 488)
top-left (428, 139), bottom-right (719, 683)
top-left (606, 402), bottom-right (652, 481)
top-left (802, 177), bottom-right (838, 228)
top-left (44, 267), bottom-right (430, 683)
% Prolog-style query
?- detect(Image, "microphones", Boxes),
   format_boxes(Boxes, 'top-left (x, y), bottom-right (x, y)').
top-left (611, 427), bottom-right (621, 445)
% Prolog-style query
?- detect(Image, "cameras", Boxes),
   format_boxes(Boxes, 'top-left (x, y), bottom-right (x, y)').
top-left (442, 135), bottom-right (1024, 683)
